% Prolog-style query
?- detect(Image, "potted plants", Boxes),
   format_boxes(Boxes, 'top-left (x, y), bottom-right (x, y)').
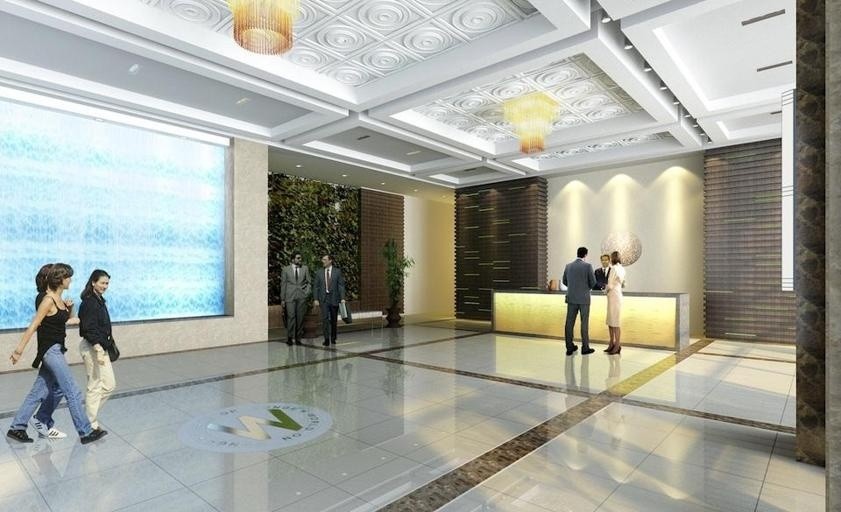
top-left (379, 240), bottom-right (414, 326)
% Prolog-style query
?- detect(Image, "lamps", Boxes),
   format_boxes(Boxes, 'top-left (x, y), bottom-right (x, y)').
top-left (501, 93), bottom-right (558, 153)
top-left (221, 1), bottom-right (301, 56)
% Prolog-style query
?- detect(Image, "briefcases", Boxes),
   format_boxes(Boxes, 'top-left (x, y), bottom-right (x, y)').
top-left (338, 302), bottom-right (352, 324)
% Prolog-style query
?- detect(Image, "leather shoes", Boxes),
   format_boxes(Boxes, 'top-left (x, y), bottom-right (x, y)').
top-left (566, 345), bottom-right (577, 356)
top-left (284, 341), bottom-right (335, 347)
top-left (581, 348), bottom-right (594, 355)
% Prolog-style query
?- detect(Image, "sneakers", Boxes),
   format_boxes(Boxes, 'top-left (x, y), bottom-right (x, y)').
top-left (80, 430), bottom-right (106, 444)
top-left (38, 427), bottom-right (66, 439)
top-left (28, 415), bottom-right (48, 437)
top-left (6, 430), bottom-right (32, 442)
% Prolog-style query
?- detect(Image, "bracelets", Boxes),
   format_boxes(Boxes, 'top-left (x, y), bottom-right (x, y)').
top-left (14, 351), bottom-right (22, 356)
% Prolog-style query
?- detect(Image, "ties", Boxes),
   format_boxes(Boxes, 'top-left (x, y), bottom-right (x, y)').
top-left (295, 267), bottom-right (297, 282)
top-left (326, 269), bottom-right (329, 291)
top-left (603, 269), bottom-right (604, 276)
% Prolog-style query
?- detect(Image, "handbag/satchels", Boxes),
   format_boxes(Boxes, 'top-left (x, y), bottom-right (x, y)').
top-left (107, 343), bottom-right (118, 361)
top-left (281, 303), bottom-right (286, 328)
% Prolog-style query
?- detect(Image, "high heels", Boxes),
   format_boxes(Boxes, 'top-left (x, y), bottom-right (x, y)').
top-left (609, 346), bottom-right (620, 355)
top-left (604, 344), bottom-right (615, 352)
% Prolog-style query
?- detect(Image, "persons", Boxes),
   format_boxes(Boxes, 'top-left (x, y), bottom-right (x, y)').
top-left (312, 253), bottom-right (346, 346)
top-left (28, 264), bottom-right (68, 439)
top-left (601, 251), bottom-right (625, 355)
top-left (78, 268), bottom-right (120, 433)
top-left (592, 255), bottom-right (612, 290)
top-left (6, 264), bottom-right (107, 444)
top-left (562, 247), bottom-right (597, 355)
top-left (280, 252), bottom-right (312, 346)
